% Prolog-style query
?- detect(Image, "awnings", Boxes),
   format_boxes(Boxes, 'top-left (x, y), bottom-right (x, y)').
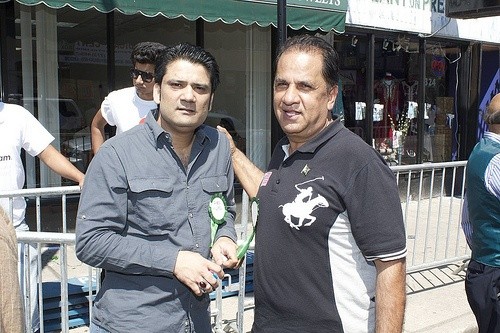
top-left (0, 0), bottom-right (348, 33)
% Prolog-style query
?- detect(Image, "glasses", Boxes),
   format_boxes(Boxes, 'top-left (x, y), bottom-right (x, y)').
top-left (130, 68), bottom-right (156, 83)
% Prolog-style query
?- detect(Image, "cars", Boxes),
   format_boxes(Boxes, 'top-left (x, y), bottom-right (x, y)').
top-left (7, 93), bottom-right (240, 187)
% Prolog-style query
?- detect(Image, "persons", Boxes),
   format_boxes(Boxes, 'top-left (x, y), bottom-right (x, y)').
top-left (0, 102), bottom-right (86, 333)
top-left (461, 93), bottom-right (500, 333)
top-left (75, 42), bottom-right (238, 333)
top-left (216, 34), bottom-right (407, 333)
top-left (91, 41), bottom-right (168, 156)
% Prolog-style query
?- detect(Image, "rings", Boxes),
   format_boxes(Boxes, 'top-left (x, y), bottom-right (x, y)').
top-left (199, 280), bottom-right (207, 290)
